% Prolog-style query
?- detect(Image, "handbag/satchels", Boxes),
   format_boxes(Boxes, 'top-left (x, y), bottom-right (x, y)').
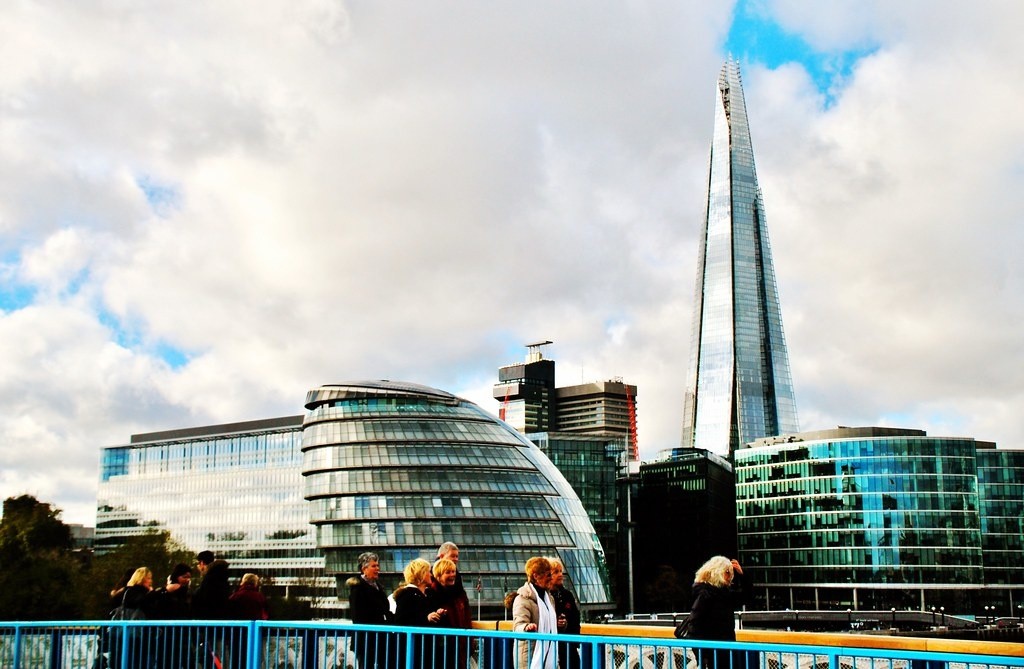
top-left (674, 591), bottom-right (706, 638)
top-left (109, 588), bottom-right (138, 620)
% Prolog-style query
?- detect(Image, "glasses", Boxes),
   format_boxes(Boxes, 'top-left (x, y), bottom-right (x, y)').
top-left (724, 570), bottom-right (730, 574)
top-left (540, 572), bottom-right (552, 577)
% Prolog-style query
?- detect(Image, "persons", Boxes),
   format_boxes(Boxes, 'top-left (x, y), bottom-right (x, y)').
top-left (346, 552), bottom-right (392, 669)
top-left (230, 573), bottom-right (269, 669)
top-left (96, 550), bottom-right (230, 669)
top-left (505, 557), bottom-right (581, 669)
top-left (387, 541), bottom-right (476, 669)
top-left (689, 556), bottom-right (752, 669)
